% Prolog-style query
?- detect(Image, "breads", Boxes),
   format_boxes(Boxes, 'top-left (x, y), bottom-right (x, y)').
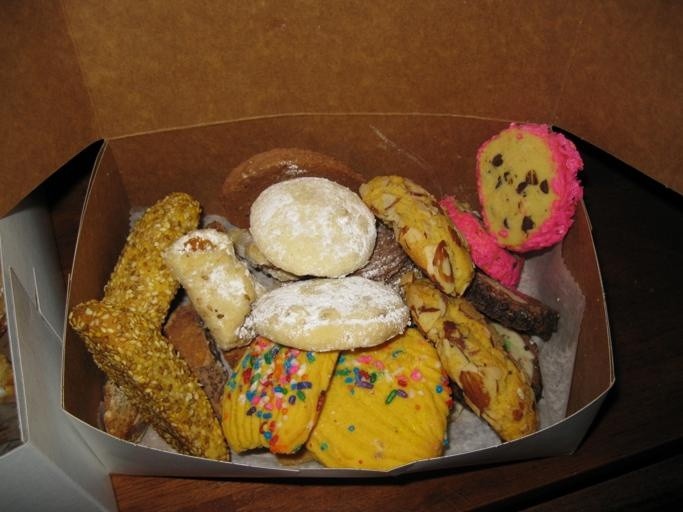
top-left (69, 122), bottom-right (593, 470)
